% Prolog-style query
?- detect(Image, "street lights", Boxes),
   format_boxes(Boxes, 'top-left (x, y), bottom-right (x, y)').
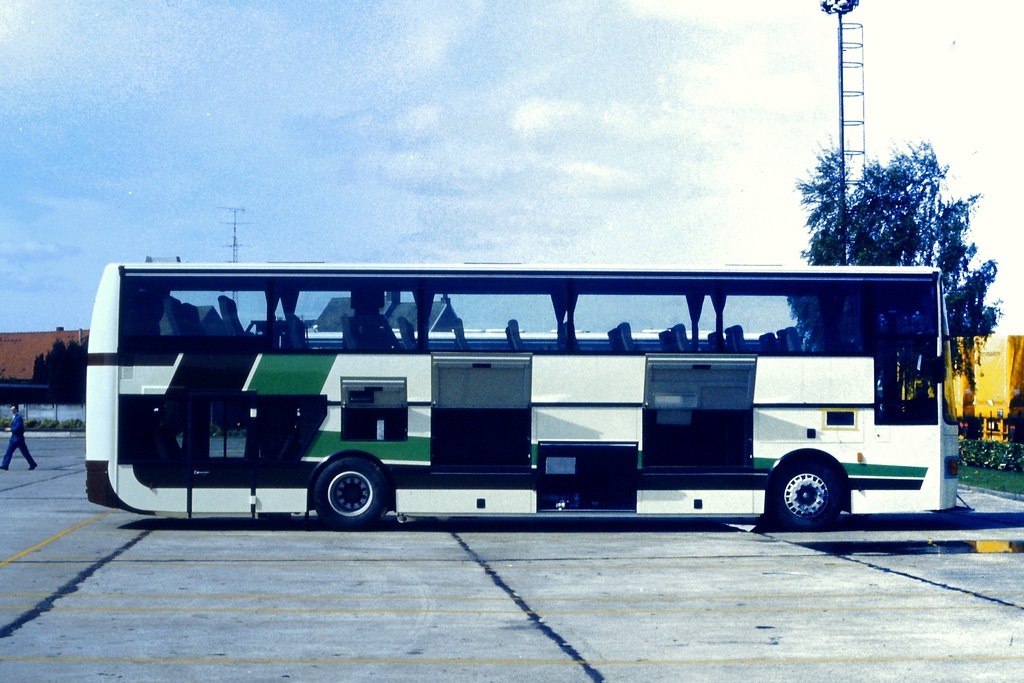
top-left (818, 0), bottom-right (859, 264)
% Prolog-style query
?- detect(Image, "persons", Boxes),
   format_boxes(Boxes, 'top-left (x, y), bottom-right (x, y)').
top-left (1009, 384), bottom-right (1024, 443)
top-left (0, 404), bottom-right (37, 471)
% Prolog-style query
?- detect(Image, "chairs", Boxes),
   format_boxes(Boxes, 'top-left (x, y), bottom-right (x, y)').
top-left (164, 295), bottom-right (803, 355)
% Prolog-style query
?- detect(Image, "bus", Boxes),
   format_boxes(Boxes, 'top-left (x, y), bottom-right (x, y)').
top-left (85, 261), bottom-right (960, 534)
